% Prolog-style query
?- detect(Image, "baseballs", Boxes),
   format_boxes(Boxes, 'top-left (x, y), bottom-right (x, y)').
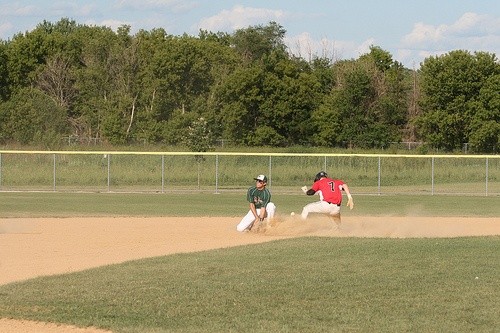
top-left (291, 212), bottom-right (294, 214)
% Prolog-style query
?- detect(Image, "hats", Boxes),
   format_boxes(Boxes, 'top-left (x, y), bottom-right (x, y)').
top-left (254, 174), bottom-right (267, 183)
top-left (314, 171), bottom-right (327, 182)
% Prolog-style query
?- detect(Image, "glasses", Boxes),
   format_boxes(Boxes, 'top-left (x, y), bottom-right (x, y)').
top-left (256, 180), bottom-right (263, 182)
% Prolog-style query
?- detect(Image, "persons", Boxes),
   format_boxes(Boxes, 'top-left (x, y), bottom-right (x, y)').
top-left (290, 170), bottom-right (355, 229)
top-left (236, 174), bottom-right (277, 233)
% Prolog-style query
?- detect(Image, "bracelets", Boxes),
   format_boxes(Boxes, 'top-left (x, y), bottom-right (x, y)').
top-left (348, 194), bottom-right (352, 199)
top-left (260, 218), bottom-right (263, 221)
